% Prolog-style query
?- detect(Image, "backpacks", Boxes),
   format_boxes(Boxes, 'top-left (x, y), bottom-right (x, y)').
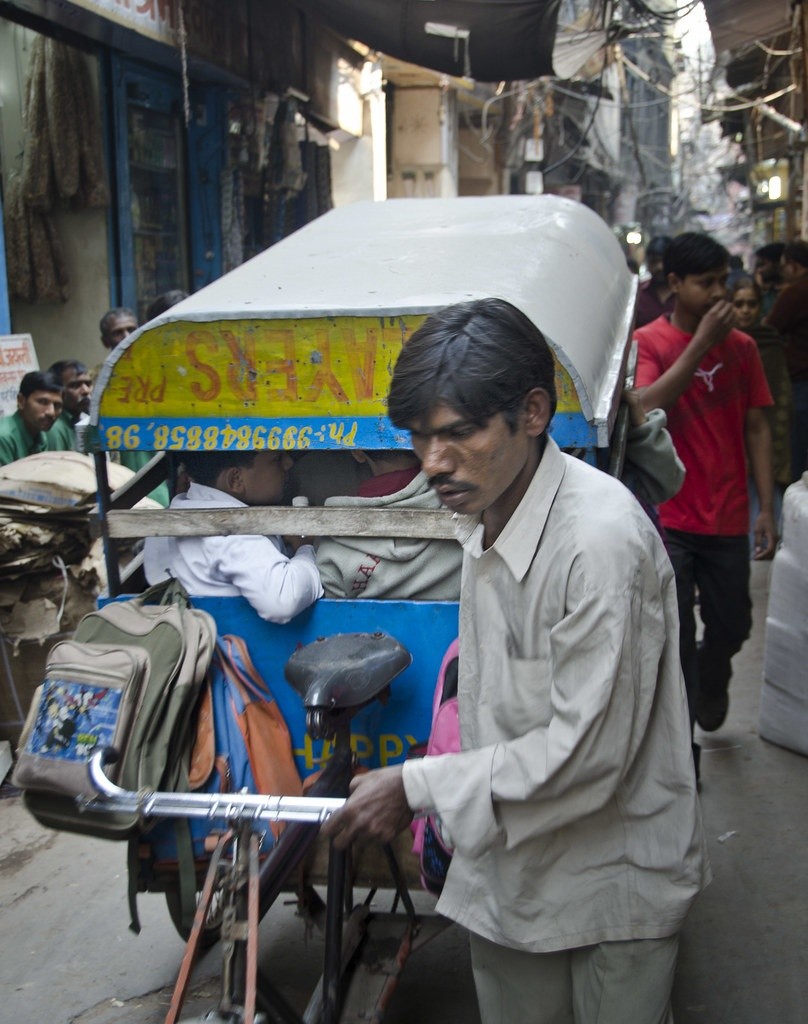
top-left (12, 577), bottom-right (213, 840)
top-left (410, 636), bottom-right (462, 900)
top-left (126, 634), bottom-right (304, 945)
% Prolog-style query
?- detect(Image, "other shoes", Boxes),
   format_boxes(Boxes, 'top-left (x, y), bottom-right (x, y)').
top-left (696, 647), bottom-right (731, 732)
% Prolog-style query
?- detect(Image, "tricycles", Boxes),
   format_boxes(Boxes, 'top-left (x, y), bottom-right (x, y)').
top-left (77, 190), bottom-right (642, 1024)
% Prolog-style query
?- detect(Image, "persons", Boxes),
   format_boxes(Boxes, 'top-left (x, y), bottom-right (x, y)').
top-left (0, 288), bottom-right (192, 509)
top-left (320, 296), bottom-right (714, 1024)
top-left (624, 232), bottom-right (808, 791)
top-left (142, 450), bottom-right (464, 625)
